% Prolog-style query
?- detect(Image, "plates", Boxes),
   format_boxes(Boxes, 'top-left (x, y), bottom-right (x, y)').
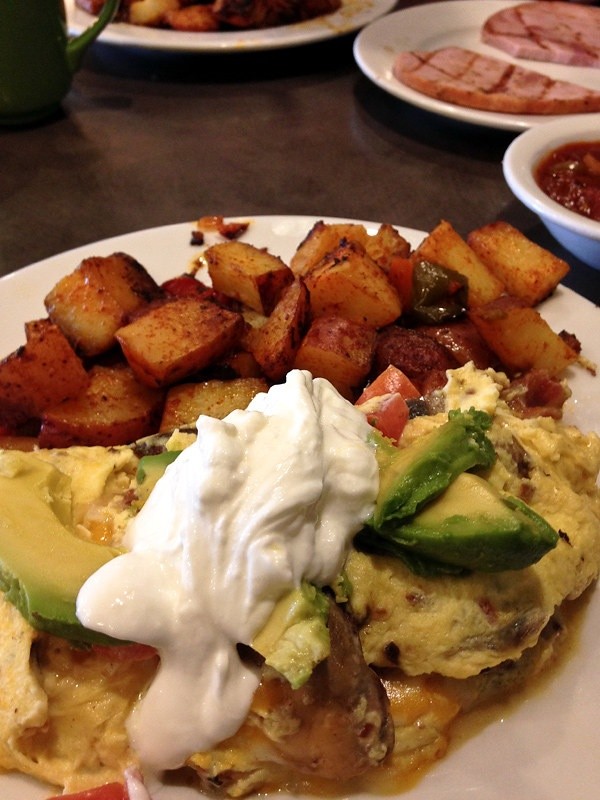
top-left (1, 214), bottom-right (600, 800)
top-left (352, 0), bottom-right (599, 134)
top-left (64, 0), bottom-right (399, 52)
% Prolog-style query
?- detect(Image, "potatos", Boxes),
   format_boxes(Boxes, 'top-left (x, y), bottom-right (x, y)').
top-left (1, 219), bottom-right (582, 446)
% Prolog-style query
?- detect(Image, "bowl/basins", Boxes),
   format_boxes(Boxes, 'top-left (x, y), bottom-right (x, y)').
top-left (502, 112), bottom-right (598, 273)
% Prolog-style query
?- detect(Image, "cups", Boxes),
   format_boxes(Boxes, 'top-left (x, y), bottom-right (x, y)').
top-left (0, 1), bottom-right (119, 131)
